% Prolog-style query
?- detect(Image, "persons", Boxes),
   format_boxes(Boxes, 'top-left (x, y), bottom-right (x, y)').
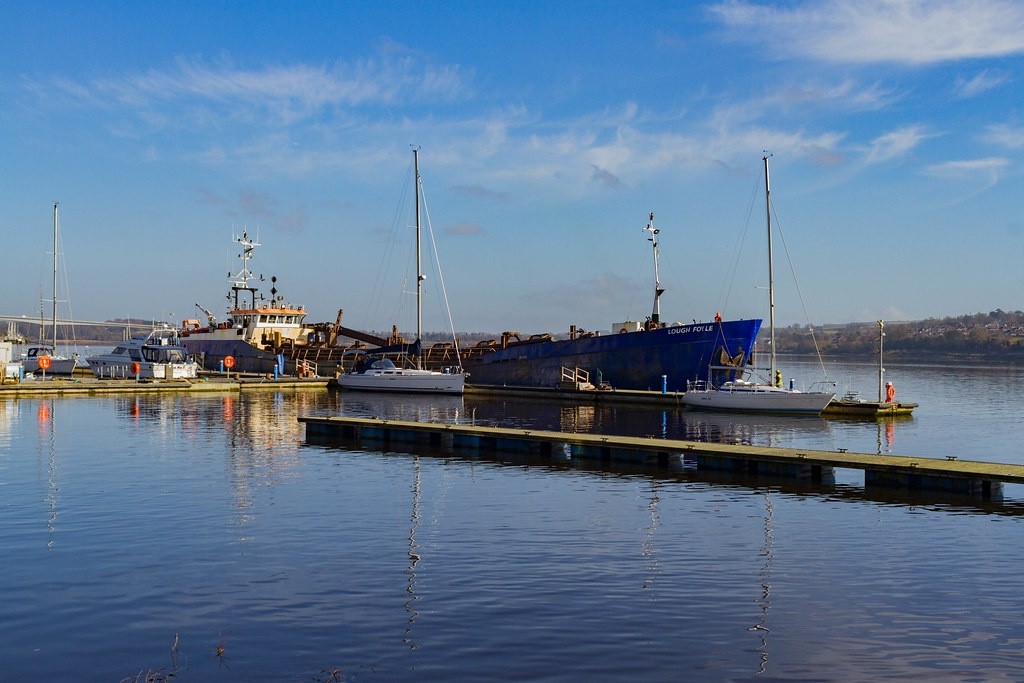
top-left (649, 320), bottom-right (657, 331)
top-left (775, 370), bottom-right (782, 387)
top-left (714, 312), bottom-right (721, 323)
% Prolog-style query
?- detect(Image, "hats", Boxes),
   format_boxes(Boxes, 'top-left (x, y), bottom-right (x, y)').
top-left (776, 369), bottom-right (780, 372)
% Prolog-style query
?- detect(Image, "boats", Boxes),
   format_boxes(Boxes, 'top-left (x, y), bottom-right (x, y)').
top-left (827, 319), bottom-right (919, 419)
top-left (178, 213), bottom-right (764, 388)
top-left (85, 307), bottom-right (198, 380)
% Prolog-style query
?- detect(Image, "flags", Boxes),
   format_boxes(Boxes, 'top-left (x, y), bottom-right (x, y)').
top-left (721, 350), bottom-right (736, 367)
top-left (724, 353), bottom-right (742, 377)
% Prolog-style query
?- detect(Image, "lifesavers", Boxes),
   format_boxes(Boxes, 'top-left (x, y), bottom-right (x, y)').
top-left (224, 355), bottom-right (235, 368)
top-left (887, 386), bottom-right (896, 398)
top-left (619, 328), bottom-right (628, 334)
top-left (130, 362), bottom-right (141, 375)
top-left (37, 355), bottom-right (51, 369)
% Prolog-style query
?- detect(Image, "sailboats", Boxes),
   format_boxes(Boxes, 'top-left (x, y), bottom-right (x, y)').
top-left (338, 142), bottom-right (468, 393)
top-left (14, 202), bottom-right (79, 375)
top-left (681, 151), bottom-right (838, 416)
